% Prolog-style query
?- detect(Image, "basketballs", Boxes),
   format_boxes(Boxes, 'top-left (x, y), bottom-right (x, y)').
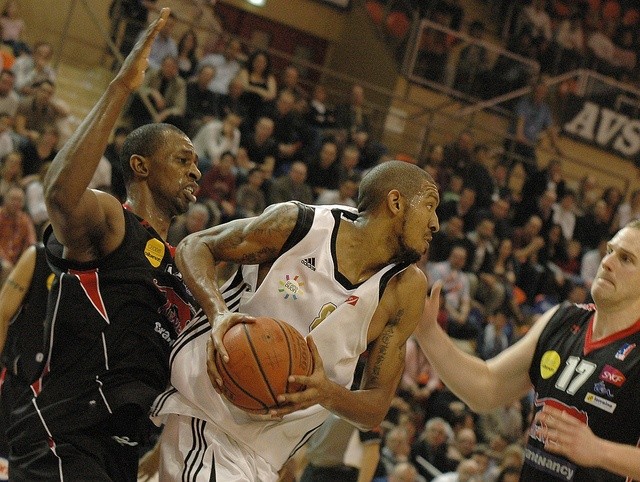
top-left (212, 316), bottom-right (313, 413)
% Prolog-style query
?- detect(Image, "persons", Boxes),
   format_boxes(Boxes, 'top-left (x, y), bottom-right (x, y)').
top-left (366, 2), bottom-right (638, 102)
top-left (1, 238), bottom-right (54, 444)
top-left (421, 71), bottom-right (640, 218)
top-left (0, 2), bottom-right (65, 262)
top-left (41, 6), bottom-right (202, 482)
top-left (432, 215), bottom-right (492, 340)
top-left (149, 157), bottom-right (441, 480)
top-left (495, 215), bottom-right (608, 301)
top-left (484, 305), bottom-right (533, 356)
top-left (411, 215), bottom-right (640, 480)
top-left (385, 377), bottom-right (531, 481)
top-left (352, 410), bottom-right (382, 481)
